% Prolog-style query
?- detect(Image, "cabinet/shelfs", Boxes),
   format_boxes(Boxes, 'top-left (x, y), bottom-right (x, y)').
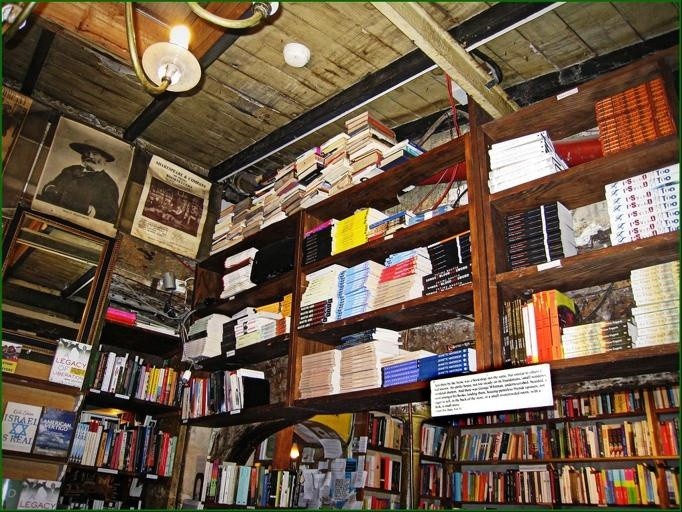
top-left (296, 2), bottom-right (499, 416)
top-left (2, 316), bottom-right (189, 510)
top-left (487, 1), bottom-right (682, 371)
top-left (179, 366), bottom-right (680, 509)
top-left (182, 106), bottom-right (321, 428)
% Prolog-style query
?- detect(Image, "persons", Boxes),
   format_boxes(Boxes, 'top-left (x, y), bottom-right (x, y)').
top-left (41, 139), bottom-right (119, 224)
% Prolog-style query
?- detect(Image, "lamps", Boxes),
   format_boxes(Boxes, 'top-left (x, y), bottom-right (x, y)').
top-left (126, 1), bottom-right (281, 95)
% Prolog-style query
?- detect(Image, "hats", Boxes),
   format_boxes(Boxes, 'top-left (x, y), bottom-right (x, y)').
top-left (68, 136), bottom-right (116, 162)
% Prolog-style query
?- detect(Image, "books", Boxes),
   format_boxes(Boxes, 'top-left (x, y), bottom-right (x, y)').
top-left (0, 339), bottom-right (179, 512)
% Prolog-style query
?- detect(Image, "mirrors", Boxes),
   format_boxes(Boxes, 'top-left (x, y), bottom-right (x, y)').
top-left (0, 204), bottom-right (122, 356)
